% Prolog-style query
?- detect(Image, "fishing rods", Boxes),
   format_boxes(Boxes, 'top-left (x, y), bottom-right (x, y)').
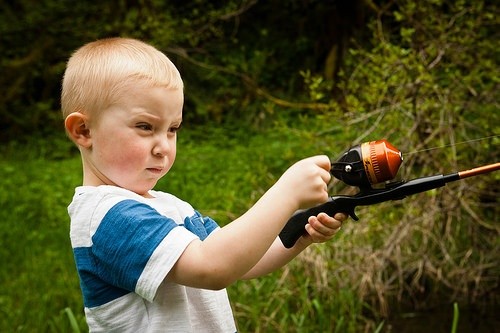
top-left (277, 139), bottom-right (500, 249)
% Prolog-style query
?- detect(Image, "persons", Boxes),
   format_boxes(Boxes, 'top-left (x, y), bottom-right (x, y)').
top-left (58, 35), bottom-right (349, 333)
